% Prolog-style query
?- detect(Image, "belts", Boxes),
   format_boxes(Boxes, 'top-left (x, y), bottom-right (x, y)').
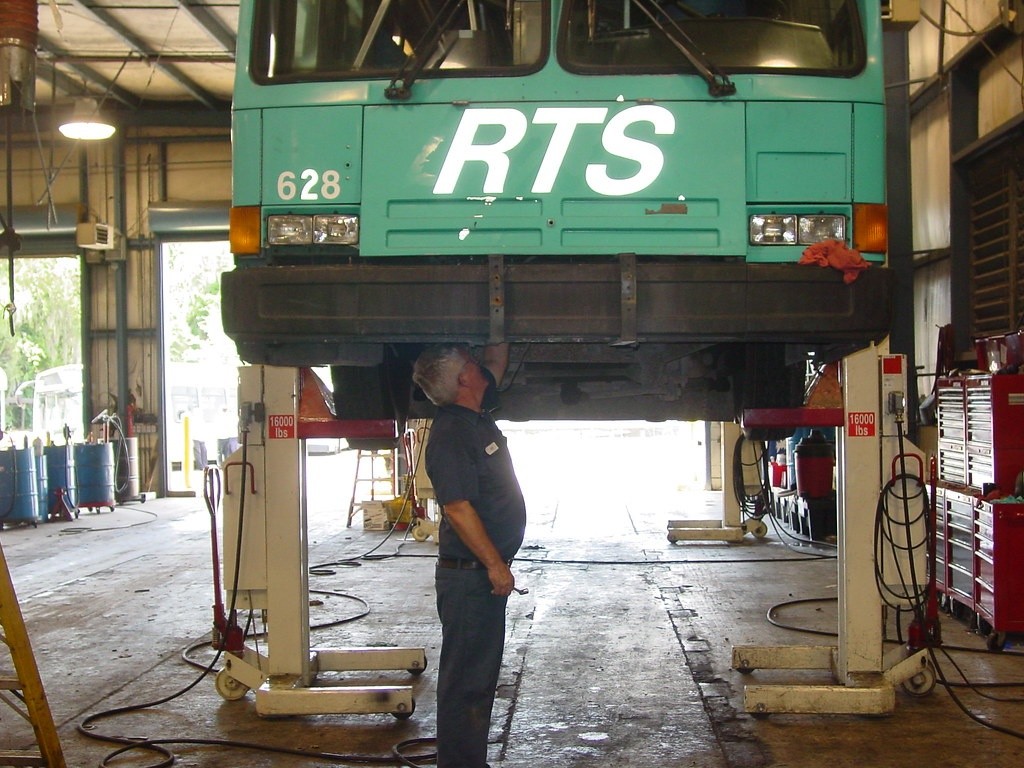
top-left (438, 556), bottom-right (514, 570)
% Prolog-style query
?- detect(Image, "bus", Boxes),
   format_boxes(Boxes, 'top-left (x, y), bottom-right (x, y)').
top-left (215, 0), bottom-right (898, 442)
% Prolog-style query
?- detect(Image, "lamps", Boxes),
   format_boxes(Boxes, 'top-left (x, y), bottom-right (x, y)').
top-left (59, 77), bottom-right (118, 140)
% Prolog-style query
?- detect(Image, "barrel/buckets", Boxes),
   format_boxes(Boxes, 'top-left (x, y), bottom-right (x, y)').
top-left (786, 426), bottom-right (834, 490)
top-left (43, 444), bottom-right (80, 519)
top-left (771, 461), bottom-right (787, 487)
top-left (73, 441), bottom-right (116, 513)
top-left (35, 454), bottom-right (50, 523)
top-left (97, 437), bottom-right (146, 506)
top-left (0, 447), bottom-right (38, 530)
top-left (792, 427), bottom-right (836, 499)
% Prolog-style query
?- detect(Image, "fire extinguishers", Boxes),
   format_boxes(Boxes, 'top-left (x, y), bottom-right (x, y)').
top-left (126, 394), bottom-right (136, 439)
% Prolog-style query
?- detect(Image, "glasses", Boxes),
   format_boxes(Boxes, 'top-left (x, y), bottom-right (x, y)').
top-left (462, 353), bottom-right (480, 368)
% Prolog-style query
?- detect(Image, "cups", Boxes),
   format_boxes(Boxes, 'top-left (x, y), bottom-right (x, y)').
top-left (975, 331), bottom-right (1024, 373)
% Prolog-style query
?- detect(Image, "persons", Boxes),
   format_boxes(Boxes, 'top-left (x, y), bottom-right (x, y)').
top-left (412, 343), bottom-right (526, 768)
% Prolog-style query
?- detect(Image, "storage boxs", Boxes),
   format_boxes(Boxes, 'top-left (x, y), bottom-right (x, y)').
top-left (361, 499), bottom-right (391, 531)
